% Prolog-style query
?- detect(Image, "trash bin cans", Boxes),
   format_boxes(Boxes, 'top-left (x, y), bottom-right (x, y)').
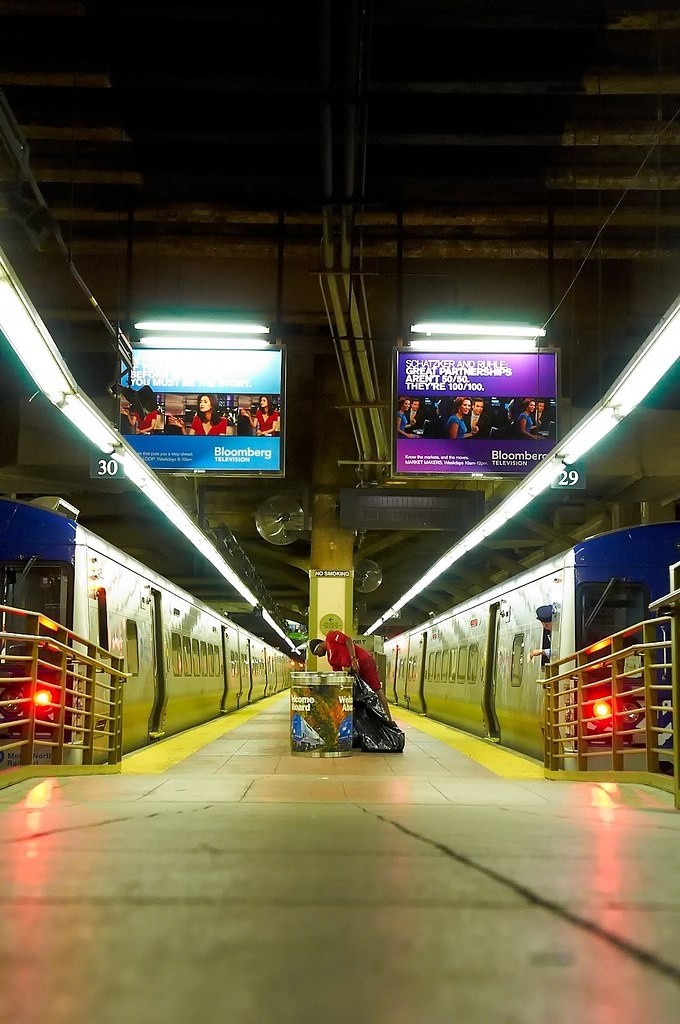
top-left (289, 670), bottom-right (355, 758)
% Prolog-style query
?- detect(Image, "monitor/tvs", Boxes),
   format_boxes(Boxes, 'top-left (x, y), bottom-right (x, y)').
top-left (115, 342), bottom-right (286, 480)
top-left (392, 346), bottom-right (562, 480)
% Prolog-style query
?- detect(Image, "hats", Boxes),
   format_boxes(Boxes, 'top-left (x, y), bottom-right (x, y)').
top-left (309, 638), bottom-right (325, 655)
top-left (536, 605), bottom-right (553, 622)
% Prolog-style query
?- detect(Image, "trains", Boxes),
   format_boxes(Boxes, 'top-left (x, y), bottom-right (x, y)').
top-left (0, 487), bottom-right (302, 766)
top-left (382, 520), bottom-right (680, 779)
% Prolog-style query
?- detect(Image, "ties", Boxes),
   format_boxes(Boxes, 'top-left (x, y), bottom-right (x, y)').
top-left (539, 413), bottom-right (542, 420)
top-left (411, 412), bottom-right (414, 422)
top-left (472, 415), bottom-right (478, 429)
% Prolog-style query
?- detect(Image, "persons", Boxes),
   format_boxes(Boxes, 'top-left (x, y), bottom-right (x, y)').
top-left (397, 396), bottom-right (551, 440)
top-left (168, 394), bottom-right (228, 435)
top-left (122, 403), bottom-right (157, 435)
top-left (240, 396), bottom-right (278, 436)
top-left (227, 415), bottom-right (233, 423)
top-left (530, 605), bottom-right (553, 661)
top-left (157, 407), bottom-right (161, 413)
top-left (310, 630), bottom-right (391, 722)
